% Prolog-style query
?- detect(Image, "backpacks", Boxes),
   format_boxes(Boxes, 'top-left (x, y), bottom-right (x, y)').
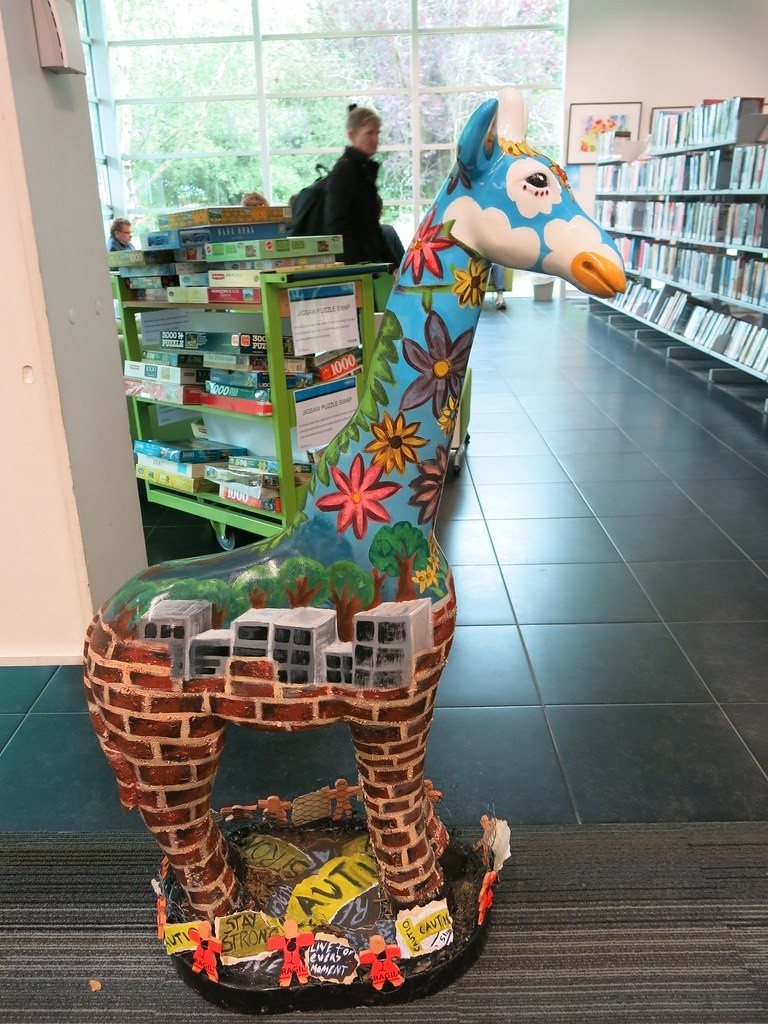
top-left (290, 162), bottom-right (331, 236)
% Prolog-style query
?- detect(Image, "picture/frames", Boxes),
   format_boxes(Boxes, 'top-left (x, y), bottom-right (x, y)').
top-left (567, 102), bottom-right (642, 165)
top-left (649, 105), bottom-right (695, 134)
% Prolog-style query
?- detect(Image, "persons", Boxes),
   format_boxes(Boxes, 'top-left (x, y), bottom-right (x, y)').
top-left (105, 218), bottom-right (136, 271)
top-left (241, 193), bottom-right (269, 207)
top-left (322, 104), bottom-right (405, 279)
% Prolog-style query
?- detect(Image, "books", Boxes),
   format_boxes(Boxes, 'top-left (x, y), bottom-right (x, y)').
top-left (591, 96), bottom-right (767, 377)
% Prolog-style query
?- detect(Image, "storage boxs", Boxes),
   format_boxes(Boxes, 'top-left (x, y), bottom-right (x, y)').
top-left (120, 262), bottom-right (224, 279)
top-left (178, 272), bottom-right (210, 287)
top-left (206, 235), bottom-right (343, 263)
top-left (125, 275), bottom-right (180, 289)
top-left (123, 329), bottom-right (362, 416)
top-left (134, 289), bottom-right (168, 301)
top-left (140, 223), bottom-right (286, 253)
top-left (109, 249), bottom-right (175, 267)
top-left (173, 247), bottom-right (206, 262)
top-left (157, 206), bottom-right (292, 233)
top-left (209, 262), bottom-right (344, 288)
top-left (167, 286), bottom-right (262, 304)
top-left (133, 439), bottom-right (328, 513)
top-left (224, 254), bottom-right (335, 271)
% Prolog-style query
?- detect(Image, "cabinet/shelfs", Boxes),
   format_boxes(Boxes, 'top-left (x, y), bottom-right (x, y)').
top-left (587, 107), bottom-right (768, 411)
top-left (110, 262), bottom-right (390, 550)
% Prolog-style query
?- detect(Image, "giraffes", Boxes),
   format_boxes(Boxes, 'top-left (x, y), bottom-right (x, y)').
top-left (82, 101), bottom-right (626, 923)
